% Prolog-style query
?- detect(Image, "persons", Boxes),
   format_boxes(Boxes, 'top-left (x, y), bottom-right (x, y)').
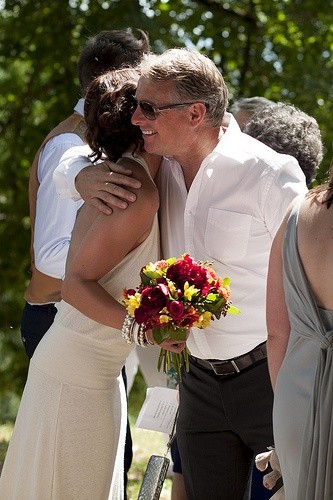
top-left (0, 68), bottom-right (190, 500)
top-left (265, 162), bottom-right (333, 500)
top-left (20, 30), bottom-right (150, 500)
top-left (233, 95), bottom-right (323, 186)
top-left (53, 48), bottom-right (308, 500)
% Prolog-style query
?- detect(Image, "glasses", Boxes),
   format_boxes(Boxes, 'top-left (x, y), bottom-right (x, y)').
top-left (131, 95), bottom-right (210, 120)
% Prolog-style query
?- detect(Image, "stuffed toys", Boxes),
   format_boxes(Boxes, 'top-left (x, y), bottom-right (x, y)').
top-left (255, 449), bottom-right (282, 490)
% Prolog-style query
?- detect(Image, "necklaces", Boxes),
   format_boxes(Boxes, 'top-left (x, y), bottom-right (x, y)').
top-left (122, 152), bottom-right (155, 186)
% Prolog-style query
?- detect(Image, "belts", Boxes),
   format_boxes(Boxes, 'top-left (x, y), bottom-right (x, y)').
top-left (189, 344), bottom-right (268, 374)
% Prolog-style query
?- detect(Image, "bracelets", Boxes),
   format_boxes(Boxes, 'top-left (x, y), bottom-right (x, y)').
top-left (122, 314), bottom-right (154, 348)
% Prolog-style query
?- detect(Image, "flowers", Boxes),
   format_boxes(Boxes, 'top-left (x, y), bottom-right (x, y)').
top-left (118, 253), bottom-right (240, 384)
